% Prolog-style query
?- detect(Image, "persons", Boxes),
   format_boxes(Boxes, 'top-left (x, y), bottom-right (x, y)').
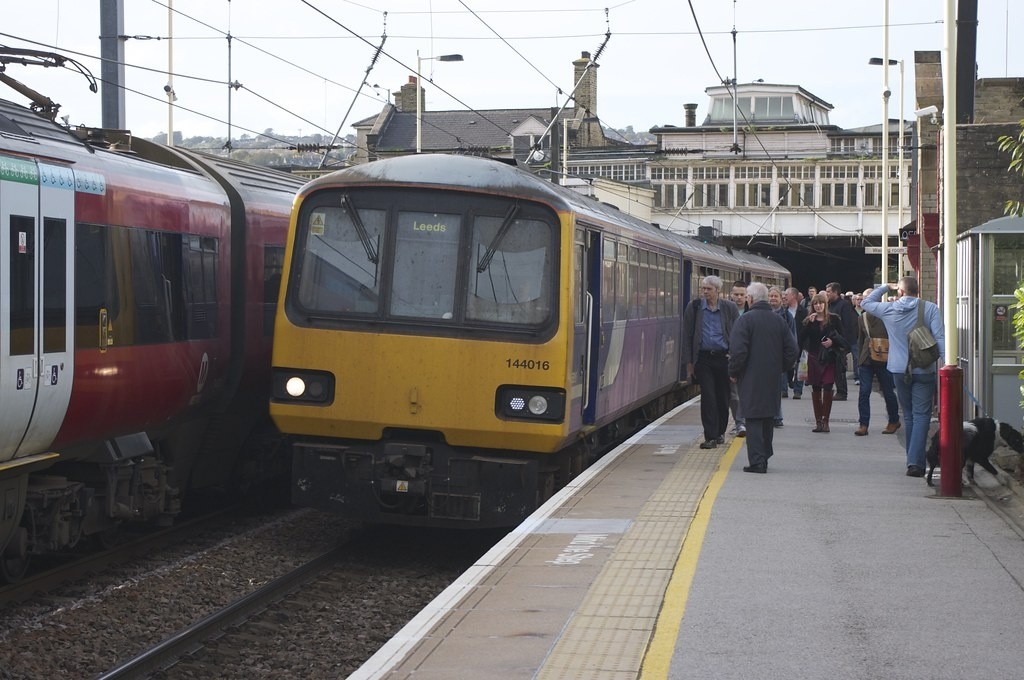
top-left (681, 275), bottom-right (946, 477)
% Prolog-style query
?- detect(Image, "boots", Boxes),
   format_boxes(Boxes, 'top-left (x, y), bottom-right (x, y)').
top-left (812, 391), bottom-right (822, 432)
top-left (820, 390), bottom-right (834, 432)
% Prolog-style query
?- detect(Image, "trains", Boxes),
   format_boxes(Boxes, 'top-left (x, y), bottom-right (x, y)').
top-left (0, 43), bottom-right (314, 590)
top-left (266, 153), bottom-right (792, 527)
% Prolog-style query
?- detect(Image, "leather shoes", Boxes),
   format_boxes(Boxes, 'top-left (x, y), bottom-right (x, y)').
top-left (743, 464), bottom-right (767, 473)
top-left (700, 439), bottom-right (717, 448)
top-left (717, 432), bottom-right (725, 444)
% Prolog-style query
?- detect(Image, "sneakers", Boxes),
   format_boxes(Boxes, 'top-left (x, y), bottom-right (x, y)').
top-left (855, 424), bottom-right (869, 435)
top-left (882, 421), bottom-right (901, 434)
top-left (736, 424), bottom-right (746, 437)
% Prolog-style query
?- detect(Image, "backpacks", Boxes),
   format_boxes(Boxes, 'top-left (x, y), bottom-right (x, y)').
top-left (903, 299), bottom-right (940, 385)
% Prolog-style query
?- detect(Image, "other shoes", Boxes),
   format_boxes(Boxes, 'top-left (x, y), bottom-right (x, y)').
top-left (855, 380), bottom-right (861, 385)
top-left (793, 392), bottom-right (802, 399)
top-left (782, 392), bottom-right (788, 397)
top-left (907, 465), bottom-right (926, 477)
top-left (789, 381), bottom-right (794, 388)
top-left (775, 420), bottom-right (783, 426)
top-left (833, 393), bottom-right (847, 401)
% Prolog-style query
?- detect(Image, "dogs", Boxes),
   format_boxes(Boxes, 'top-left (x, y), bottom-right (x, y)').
top-left (926, 417), bottom-right (1024, 486)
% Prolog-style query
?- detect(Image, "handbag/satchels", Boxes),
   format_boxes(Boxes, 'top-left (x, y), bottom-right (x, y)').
top-left (868, 338), bottom-right (889, 362)
top-left (797, 349), bottom-right (809, 381)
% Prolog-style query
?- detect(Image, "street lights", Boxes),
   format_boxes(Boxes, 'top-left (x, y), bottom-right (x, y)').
top-left (868, 57), bottom-right (905, 283)
top-left (416, 49), bottom-right (463, 155)
